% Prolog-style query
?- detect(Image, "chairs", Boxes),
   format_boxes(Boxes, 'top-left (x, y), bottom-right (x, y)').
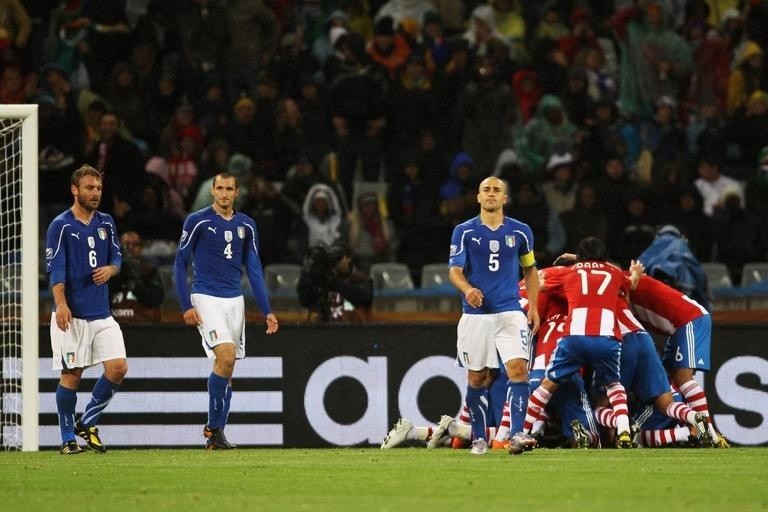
top-left (134, 262), bottom-right (768, 316)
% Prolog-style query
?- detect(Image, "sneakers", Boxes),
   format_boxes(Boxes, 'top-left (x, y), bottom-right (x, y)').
top-left (510, 431), bottom-right (536, 453)
top-left (471, 438), bottom-right (489, 453)
top-left (74, 418), bottom-right (106, 452)
top-left (60, 441), bottom-right (86, 454)
top-left (617, 433), bottom-right (633, 448)
top-left (203, 425), bottom-right (231, 449)
top-left (206, 440), bottom-right (237, 450)
top-left (426, 416), bottom-right (456, 450)
top-left (695, 412), bottom-right (712, 447)
top-left (715, 437), bottom-right (727, 448)
top-left (492, 439), bottom-right (510, 450)
top-left (381, 418), bottom-right (413, 450)
top-left (570, 419), bottom-right (589, 449)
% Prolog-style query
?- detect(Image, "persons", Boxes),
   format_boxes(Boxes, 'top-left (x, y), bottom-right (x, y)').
top-left (169, 173), bottom-right (280, 452)
top-left (42, 164), bottom-right (130, 454)
top-left (449, 176), bottom-right (538, 454)
top-left (380, 238), bottom-right (730, 454)
top-left (299, 245), bottom-right (376, 325)
top-left (2, 1), bottom-right (767, 287)
top-left (104, 230), bottom-right (167, 328)
